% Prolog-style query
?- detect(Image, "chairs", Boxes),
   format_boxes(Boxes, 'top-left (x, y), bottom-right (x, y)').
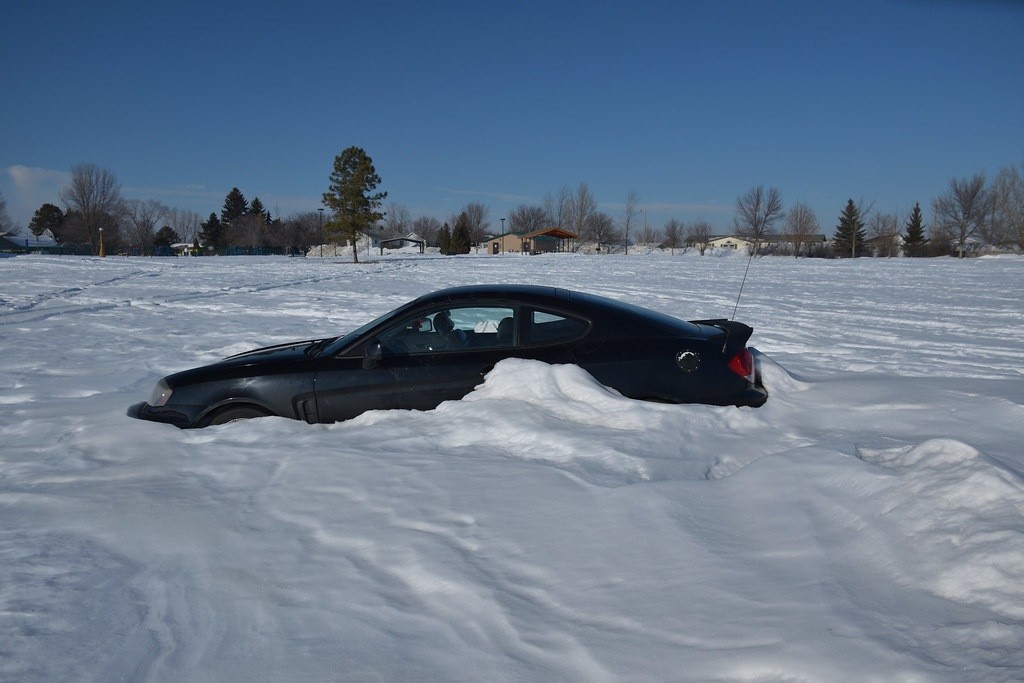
top-left (432, 314), bottom-right (470, 348)
top-left (496, 317), bottom-right (513, 347)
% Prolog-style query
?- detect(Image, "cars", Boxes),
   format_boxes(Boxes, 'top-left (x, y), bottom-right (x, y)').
top-left (141, 285), bottom-right (767, 429)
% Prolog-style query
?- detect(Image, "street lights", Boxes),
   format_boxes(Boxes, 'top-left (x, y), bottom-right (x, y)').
top-left (501, 219), bottom-right (505, 255)
top-left (318, 209), bottom-right (324, 257)
